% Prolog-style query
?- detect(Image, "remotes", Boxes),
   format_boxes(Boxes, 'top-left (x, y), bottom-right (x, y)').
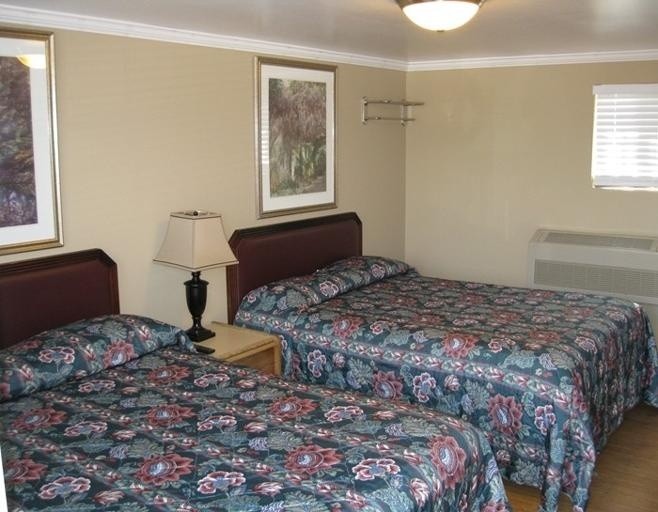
top-left (193, 343), bottom-right (215, 353)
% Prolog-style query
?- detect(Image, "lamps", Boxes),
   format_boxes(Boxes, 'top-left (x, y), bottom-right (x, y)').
top-left (154, 209), bottom-right (239, 343)
top-left (395, 0), bottom-right (485, 32)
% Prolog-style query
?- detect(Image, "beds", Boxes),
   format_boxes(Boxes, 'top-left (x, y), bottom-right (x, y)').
top-left (0, 248), bottom-right (480, 512)
top-left (226, 211), bottom-right (645, 491)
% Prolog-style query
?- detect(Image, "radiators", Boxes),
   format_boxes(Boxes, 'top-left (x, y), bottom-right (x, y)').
top-left (526, 228), bottom-right (658, 306)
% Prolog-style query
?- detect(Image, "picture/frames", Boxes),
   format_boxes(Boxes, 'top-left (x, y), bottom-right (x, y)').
top-left (0, 20), bottom-right (64, 256)
top-left (253, 55), bottom-right (337, 219)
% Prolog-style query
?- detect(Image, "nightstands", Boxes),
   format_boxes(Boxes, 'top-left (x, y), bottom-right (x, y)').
top-left (193, 321), bottom-right (281, 378)
